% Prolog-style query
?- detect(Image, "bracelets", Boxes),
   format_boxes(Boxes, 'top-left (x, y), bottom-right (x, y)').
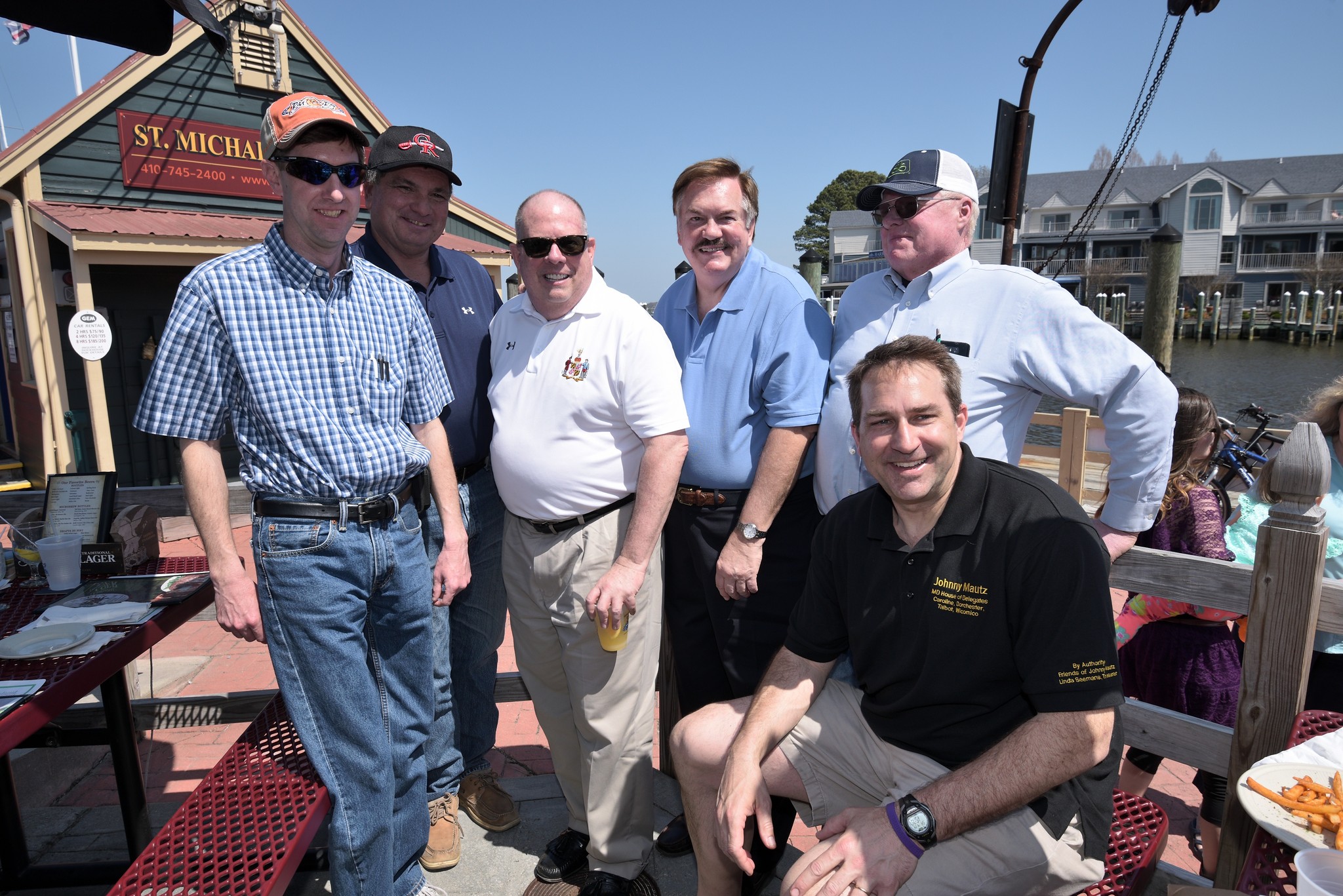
top-left (885, 802), bottom-right (925, 859)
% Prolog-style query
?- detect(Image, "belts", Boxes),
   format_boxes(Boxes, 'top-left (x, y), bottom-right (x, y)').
top-left (459, 460), bottom-right (490, 482)
top-left (677, 486), bottom-right (751, 509)
top-left (503, 494), bottom-right (635, 536)
top-left (253, 476), bottom-right (423, 524)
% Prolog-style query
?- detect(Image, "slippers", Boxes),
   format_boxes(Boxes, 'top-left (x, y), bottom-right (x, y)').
top-left (1190, 818), bottom-right (1204, 858)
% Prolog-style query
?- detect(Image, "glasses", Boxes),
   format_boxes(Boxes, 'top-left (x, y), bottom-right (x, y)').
top-left (514, 234), bottom-right (588, 258)
top-left (869, 196), bottom-right (967, 228)
top-left (268, 154), bottom-right (370, 189)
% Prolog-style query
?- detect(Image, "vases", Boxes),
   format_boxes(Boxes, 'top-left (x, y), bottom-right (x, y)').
top-left (1206, 306), bottom-right (1213, 314)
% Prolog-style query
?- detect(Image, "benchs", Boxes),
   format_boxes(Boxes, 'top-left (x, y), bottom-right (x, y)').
top-left (104, 688), bottom-right (336, 896)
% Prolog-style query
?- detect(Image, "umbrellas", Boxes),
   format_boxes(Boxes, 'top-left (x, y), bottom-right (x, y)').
top-left (0, 0), bottom-right (228, 57)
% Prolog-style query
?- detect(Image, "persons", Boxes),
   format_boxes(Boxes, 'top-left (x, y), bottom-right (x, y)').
top-left (1094, 375), bottom-right (1343, 896)
top-left (132, 92), bottom-right (473, 896)
top-left (668, 334), bottom-right (1128, 896)
top-left (490, 189), bottom-right (694, 896)
top-left (648, 157), bottom-right (835, 892)
top-left (343, 126), bottom-right (529, 874)
top-left (814, 148), bottom-right (1180, 688)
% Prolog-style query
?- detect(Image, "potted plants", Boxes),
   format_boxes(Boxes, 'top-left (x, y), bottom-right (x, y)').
top-left (1182, 302), bottom-right (1191, 310)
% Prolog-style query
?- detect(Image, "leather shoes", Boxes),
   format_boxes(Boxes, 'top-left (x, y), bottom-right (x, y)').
top-left (655, 814), bottom-right (697, 857)
top-left (739, 861), bottom-right (779, 896)
top-left (535, 829), bottom-right (592, 884)
top-left (573, 871), bottom-right (630, 896)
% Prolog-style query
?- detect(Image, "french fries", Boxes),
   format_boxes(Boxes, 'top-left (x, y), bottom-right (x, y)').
top-left (1246, 770), bottom-right (1343, 852)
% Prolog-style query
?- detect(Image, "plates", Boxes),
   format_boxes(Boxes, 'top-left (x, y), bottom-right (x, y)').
top-left (1235, 764), bottom-right (1343, 851)
top-left (161, 576), bottom-right (180, 593)
top-left (0, 622), bottom-right (96, 658)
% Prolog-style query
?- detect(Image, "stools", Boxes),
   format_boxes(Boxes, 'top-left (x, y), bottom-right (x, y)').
top-left (1071, 788), bottom-right (1173, 896)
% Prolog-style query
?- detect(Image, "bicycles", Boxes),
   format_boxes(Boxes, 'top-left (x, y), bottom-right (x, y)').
top-left (1192, 402), bottom-right (1290, 524)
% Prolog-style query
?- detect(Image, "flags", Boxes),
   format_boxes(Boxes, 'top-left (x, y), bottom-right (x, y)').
top-left (3, 19), bottom-right (34, 45)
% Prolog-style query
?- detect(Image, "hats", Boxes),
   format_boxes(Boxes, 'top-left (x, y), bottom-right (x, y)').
top-left (857, 148), bottom-right (978, 210)
top-left (262, 92), bottom-right (370, 154)
top-left (365, 125), bottom-right (462, 188)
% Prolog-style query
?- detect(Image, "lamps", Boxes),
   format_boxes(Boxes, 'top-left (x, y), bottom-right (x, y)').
top-left (254, 5), bottom-right (285, 35)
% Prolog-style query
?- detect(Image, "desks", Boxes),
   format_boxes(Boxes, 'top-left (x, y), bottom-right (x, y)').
top-left (0, 555), bottom-right (246, 896)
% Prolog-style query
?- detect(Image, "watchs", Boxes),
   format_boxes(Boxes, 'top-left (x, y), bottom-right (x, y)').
top-left (735, 519), bottom-right (768, 541)
top-left (897, 793), bottom-right (937, 852)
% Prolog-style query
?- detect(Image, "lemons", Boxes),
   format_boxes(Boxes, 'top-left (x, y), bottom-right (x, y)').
top-left (15, 548), bottom-right (41, 561)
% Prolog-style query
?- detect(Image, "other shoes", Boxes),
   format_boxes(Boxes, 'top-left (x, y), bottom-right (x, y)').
top-left (461, 767), bottom-right (522, 833)
top-left (418, 792), bottom-right (464, 871)
top-left (411, 882), bottom-right (449, 896)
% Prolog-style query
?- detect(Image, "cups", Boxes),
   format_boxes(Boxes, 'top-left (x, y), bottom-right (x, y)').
top-left (0, 542), bottom-right (7, 581)
top-left (594, 601), bottom-right (629, 651)
top-left (35, 534), bottom-right (83, 591)
top-left (1294, 848), bottom-right (1342, 896)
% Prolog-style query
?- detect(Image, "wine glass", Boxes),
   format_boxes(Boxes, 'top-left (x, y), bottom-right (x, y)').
top-left (10, 522), bottom-right (50, 587)
